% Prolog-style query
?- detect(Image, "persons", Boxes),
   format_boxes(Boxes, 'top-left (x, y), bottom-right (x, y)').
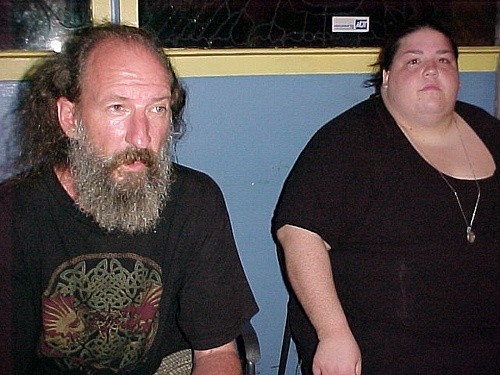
top-left (271, 16), bottom-right (500, 375)
top-left (0, 20), bottom-right (260, 375)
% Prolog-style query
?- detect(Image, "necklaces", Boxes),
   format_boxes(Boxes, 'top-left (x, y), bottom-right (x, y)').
top-left (399, 111), bottom-right (480, 245)
